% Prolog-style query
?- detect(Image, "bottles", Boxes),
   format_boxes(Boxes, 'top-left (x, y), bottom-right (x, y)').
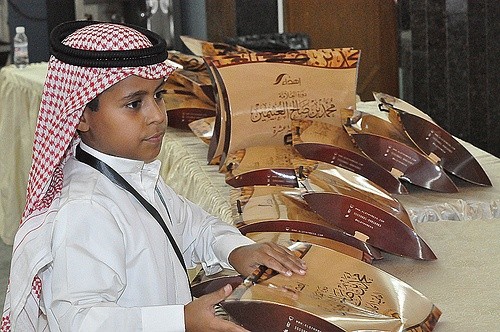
top-left (14, 26), bottom-right (29, 68)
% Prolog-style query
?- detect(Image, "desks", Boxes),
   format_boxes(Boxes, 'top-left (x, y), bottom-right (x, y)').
top-left (0, 61), bottom-right (500, 332)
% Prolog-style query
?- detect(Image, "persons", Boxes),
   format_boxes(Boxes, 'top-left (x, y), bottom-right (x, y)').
top-left (0, 21), bottom-right (306, 332)
